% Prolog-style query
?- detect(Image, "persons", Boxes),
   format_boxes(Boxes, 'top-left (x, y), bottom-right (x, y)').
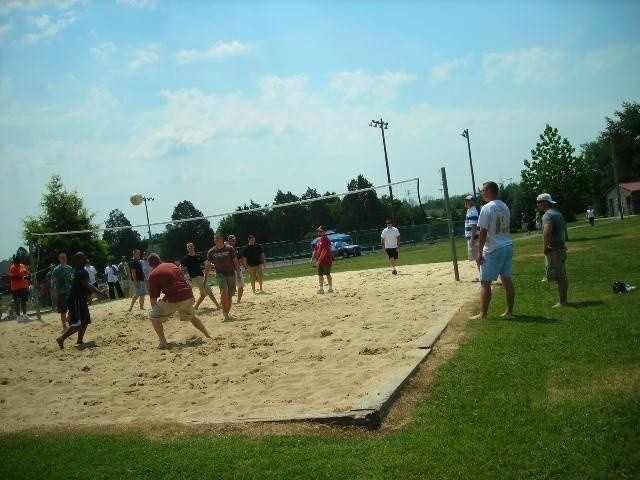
top-left (243, 233), bottom-right (266, 295)
top-left (464, 194), bottom-right (482, 282)
top-left (203, 232), bottom-right (241, 323)
top-left (586, 206), bottom-right (596, 226)
top-left (8, 248), bottom-right (153, 350)
top-left (536, 193), bottom-right (570, 308)
top-left (146, 252), bottom-right (211, 349)
top-left (381, 219), bottom-right (400, 275)
top-left (521, 211), bottom-right (531, 237)
top-left (227, 235), bottom-right (244, 304)
top-left (467, 181), bottom-right (515, 321)
top-left (174, 242), bottom-right (223, 311)
top-left (532, 207), bottom-right (543, 234)
top-left (310, 225), bottom-right (333, 294)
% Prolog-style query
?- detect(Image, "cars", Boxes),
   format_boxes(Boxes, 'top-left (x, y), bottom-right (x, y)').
top-left (331, 239), bottom-right (362, 260)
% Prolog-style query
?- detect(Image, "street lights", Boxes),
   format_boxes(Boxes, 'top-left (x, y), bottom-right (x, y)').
top-left (142, 195), bottom-right (157, 258)
top-left (366, 116), bottom-right (399, 230)
top-left (460, 125), bottom-right (479, 209)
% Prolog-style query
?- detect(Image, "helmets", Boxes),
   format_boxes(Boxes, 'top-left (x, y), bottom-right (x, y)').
top-left (536, 192), bottom-right (557, 205)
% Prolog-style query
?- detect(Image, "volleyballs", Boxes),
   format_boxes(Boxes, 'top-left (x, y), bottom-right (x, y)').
top-left (130, 194), bottom-right (143, 205)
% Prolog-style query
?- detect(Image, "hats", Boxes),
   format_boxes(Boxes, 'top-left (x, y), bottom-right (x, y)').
top-left (462, 195), bottom-right (475, 201)
top-left (315, 225), bottom-right (328, 232)
top-left (227, 234), bottom-right (236, 241)
